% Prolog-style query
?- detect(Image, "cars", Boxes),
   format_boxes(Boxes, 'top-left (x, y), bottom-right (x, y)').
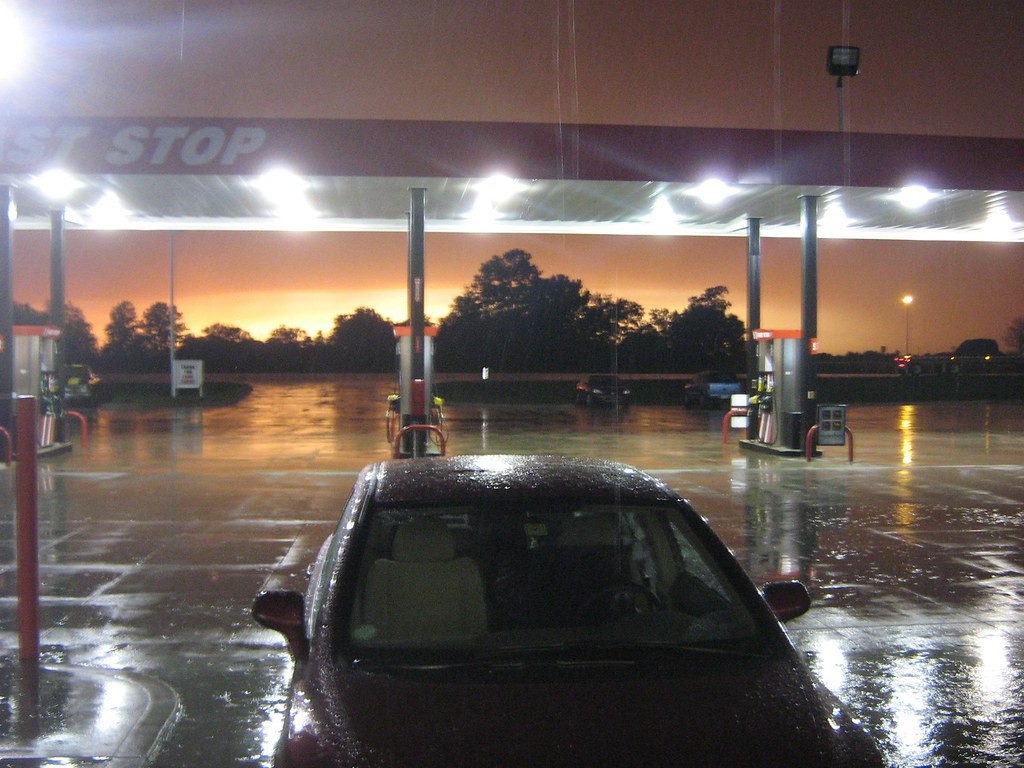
top-left (899, 358), bottom-right (942, 375)
top-left (250, 454), bottom-right (886, 768)
top-left (683, 371), bottom-right (742, 409)
top-left (64, 365), bottom-right (102, 407)
top-left (576, 374), bottom-right (631, 409)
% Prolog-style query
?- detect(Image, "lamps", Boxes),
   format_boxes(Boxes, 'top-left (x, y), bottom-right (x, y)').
top-left (827, 43), bottom-right (862, 85)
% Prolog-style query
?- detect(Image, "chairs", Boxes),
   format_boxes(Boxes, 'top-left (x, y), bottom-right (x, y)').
top-left (363, 517), bottom-right (488, 645)
top-left (553, 511), bottom-right (657, 630)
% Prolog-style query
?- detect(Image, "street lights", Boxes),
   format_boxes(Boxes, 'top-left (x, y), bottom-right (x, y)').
top-left (903, 294), bottom-right (914, 355)
